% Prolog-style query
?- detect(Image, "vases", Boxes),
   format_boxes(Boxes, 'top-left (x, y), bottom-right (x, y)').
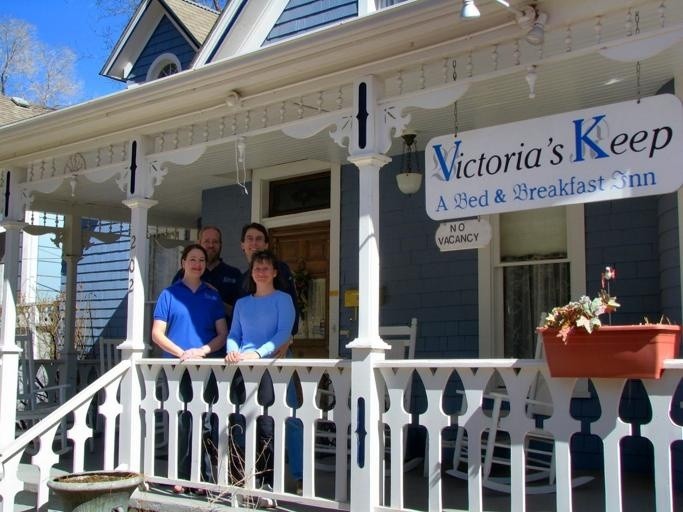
top-left (535, 324), bottom-right (681, 379)
top-left (47, 470), bottom-right (145, 512)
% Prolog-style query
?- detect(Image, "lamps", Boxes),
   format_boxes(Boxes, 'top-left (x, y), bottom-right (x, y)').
top-left (462, 0), bottom-right (548, 46)
top-left (225, 92), bottom-right (242, 111)
top-left (396, 135), bottom-right (423, 197)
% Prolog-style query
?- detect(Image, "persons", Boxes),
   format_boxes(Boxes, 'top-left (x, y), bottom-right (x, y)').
top-left (150, 243), bottom-right (228, 498)
top-left (229, 222), bottom-right (304, 495)
top-left (226, 248), bottom-right (297, 510)
top-left (170, 224), bottom-right (242, 332)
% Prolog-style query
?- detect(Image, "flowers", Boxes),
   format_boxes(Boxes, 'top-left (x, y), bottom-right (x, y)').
top-left (544, 266), bottom-right (621, 344)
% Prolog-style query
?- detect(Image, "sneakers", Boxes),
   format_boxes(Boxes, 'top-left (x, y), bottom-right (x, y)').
top-left (174, 486), bottom-right (203, 494)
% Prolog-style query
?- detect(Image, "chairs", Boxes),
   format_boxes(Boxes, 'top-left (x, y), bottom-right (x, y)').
top-left (317, 320), bottom-right (419, 481)
top-left (16, 333), bottom-right (169, 456)
top-left (446, 321), bottom-right (594, 493)
